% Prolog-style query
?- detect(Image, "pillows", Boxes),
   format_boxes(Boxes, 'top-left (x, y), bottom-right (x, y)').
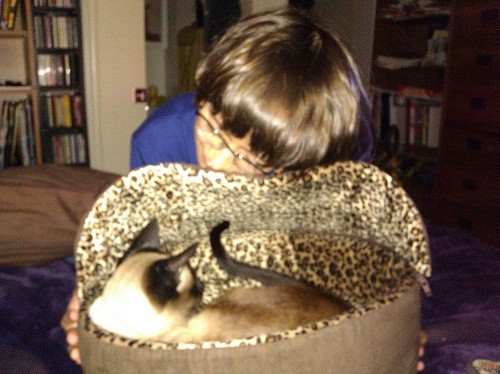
top-left (1, 163), bottom-right (122, 269)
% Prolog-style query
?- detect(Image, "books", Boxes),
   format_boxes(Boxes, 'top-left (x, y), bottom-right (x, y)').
top-left (374, 89), bottom-right (442, 149)
top-left (0, 0), bottom-right (89, 168)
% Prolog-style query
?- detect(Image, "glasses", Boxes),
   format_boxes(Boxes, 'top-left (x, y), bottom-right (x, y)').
top-left (194, 99), bottom-right (278, 176)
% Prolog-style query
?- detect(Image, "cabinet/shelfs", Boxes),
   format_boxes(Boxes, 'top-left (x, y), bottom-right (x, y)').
top-left (364, 1), bottom-right (500, 247)
top-left (1, 1), bottom-right (92, 170)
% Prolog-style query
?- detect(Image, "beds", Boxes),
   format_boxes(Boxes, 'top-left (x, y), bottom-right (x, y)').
top-left (0, 166), bottom-right (500, 373)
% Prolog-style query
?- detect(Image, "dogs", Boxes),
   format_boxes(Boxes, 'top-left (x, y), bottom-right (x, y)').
top-left (89, 216), bottom-right (354, 343)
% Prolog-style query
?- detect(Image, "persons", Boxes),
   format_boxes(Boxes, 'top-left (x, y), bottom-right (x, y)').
top-left (61, 7), bottom-right (427, 374)
